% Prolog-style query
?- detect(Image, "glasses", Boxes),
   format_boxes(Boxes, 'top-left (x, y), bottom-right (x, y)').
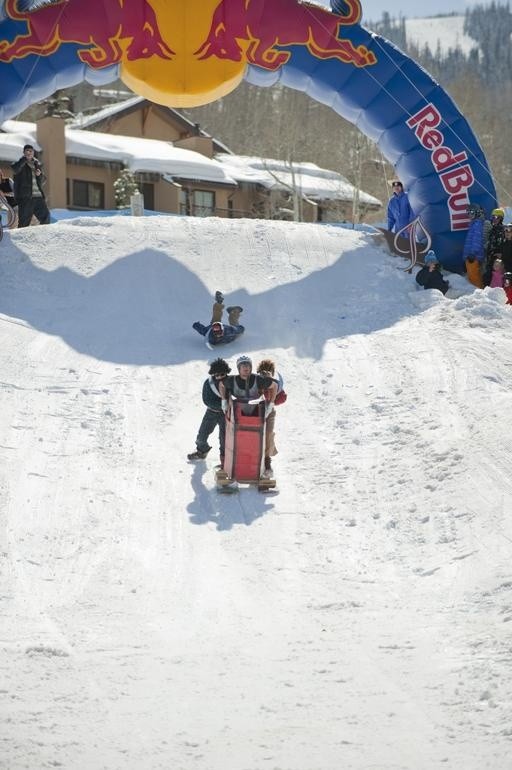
top-left (214, 330), bottom-right (223, 335)
top-left (214, 374), bottom-right (225, 377)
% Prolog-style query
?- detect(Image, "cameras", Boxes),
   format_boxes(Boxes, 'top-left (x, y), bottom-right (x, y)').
top-left (435, 263), bottom-right (440, 270)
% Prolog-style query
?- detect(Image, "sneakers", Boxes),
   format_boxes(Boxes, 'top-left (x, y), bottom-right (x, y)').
top-left (215, 291), bottom-right (224, 304)
top-left (226, 306), bottom-right (243, 314)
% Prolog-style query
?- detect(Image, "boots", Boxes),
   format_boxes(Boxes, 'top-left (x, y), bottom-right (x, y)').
top-left (187, 445), bottom-right (212, 460)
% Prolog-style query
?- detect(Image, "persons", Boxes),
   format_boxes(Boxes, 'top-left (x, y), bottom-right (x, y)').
top-left (414, 249), bottom-right (450, 297)
top-left (255, 357), bottom-right (288, 479)
top-left (387, 180), bottom-right (418, 241)
top-left (192, 290), bottom-right (245, 345)
top-left (501, 223), bottom-right (512, 274)
top-left (479, 207), bottom-right (494, 287)
top-left (486, 208), bottom-right (507, 286)
top-left (502, 271), bottom-right (512, 306)
top-left (489, 258), bottom-right (506, 288)
top-left (8, 144), bottom-right (51, 229)
top-left (185, 356), bottom-right (230, 473)
top-left (0, 170), bottom-right (39, 227)
top-left (217, 355), bottom-right (280, 418)
top-left (462, 202), bottom-right (487, 289)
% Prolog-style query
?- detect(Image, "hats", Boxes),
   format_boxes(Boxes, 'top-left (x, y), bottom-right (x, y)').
top-left (386, 175), bottom-right (402, 186)
top-left (423, 250), bottom-right (439, 266)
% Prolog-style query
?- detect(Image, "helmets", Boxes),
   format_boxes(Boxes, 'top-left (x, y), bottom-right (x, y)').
top-left (466, 204), bottom-right (484, 214)
top-left (491, 209), bottom-right (504, 218)
top-left (236, 355), bottom-right (253, 367)
